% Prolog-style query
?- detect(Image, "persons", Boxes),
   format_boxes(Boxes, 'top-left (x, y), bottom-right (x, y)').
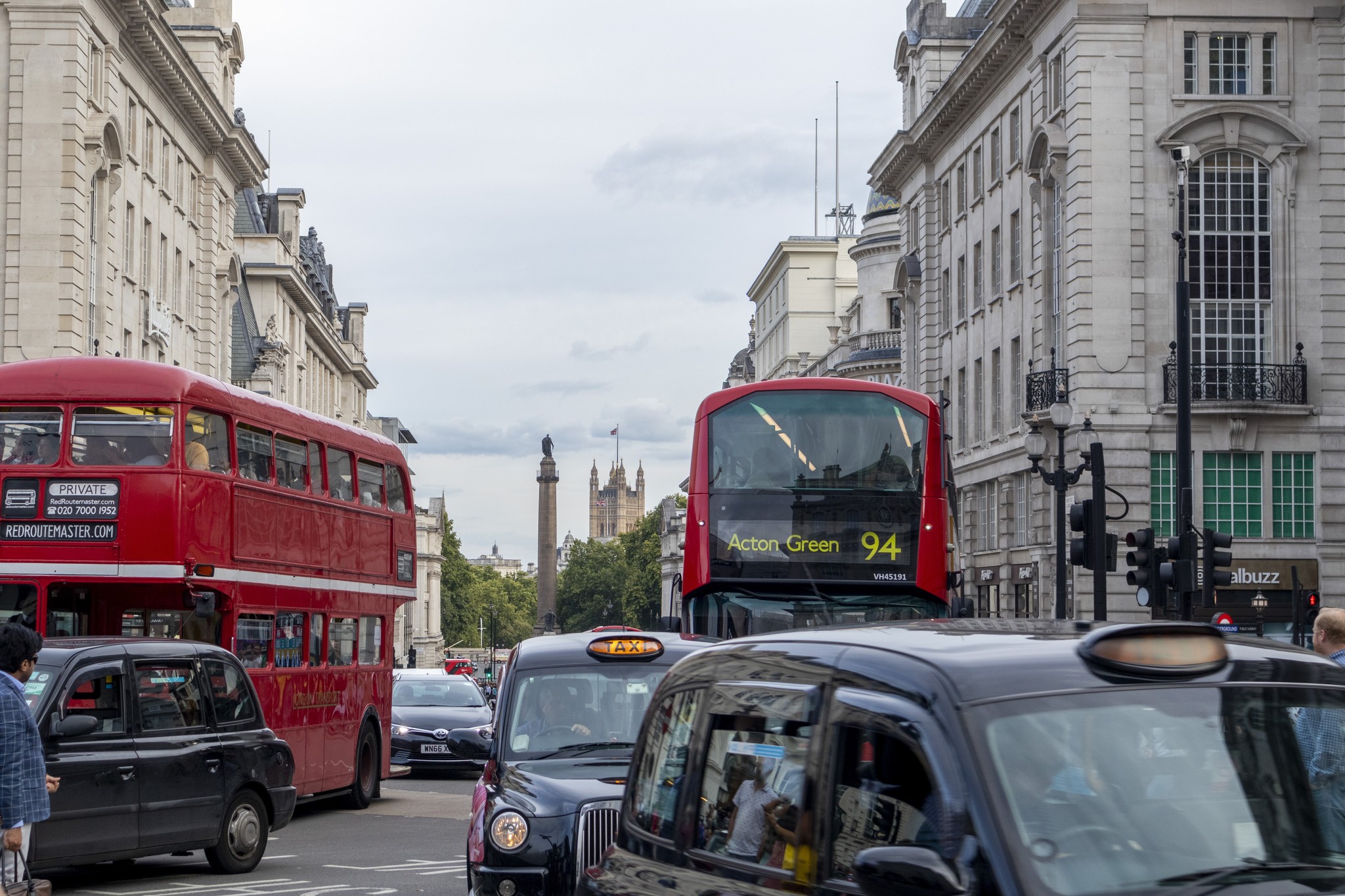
top-left (171, 421), bottom-right (209, 472)
top-left (0, 621), bottom-right (61, 896)
top-left (241, 645), bottom-right (263, 669)
top-left (78, 437), bottom-right (122, 466)
top-left (0, 428), bottom-right (39, 465)
top-left (217, 447), bottom-right (406, 513)
top-left (101, 434), bottom-right (166, 467)
top-left (33, 431), bottom-right (62, 464)
top-left (515, 682), bottom-right (596, 747)
top-left (479, 681), bottom-right (497, 701)
top-left (1295, 607), bottom-right (1345, 855)
top-left (398, 685), bottom-right (414, 704)
top-left (721, 754), bottom-right (801, 873)
top-left (1061, 711), bottom-right (1215, 869)
top-left (541, 434), bottom-right (554, 456)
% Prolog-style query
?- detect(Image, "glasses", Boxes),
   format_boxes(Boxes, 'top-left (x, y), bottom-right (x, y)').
top-left (756, 761), bottom-right (770, 771)
top-left (33, 655), bottom-right (39, 664)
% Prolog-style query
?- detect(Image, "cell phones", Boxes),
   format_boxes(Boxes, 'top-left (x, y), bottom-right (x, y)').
top-left (760, 803), bottom-right (771, 813)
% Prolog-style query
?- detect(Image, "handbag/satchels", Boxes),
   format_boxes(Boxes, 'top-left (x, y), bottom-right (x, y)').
top-left (708, 818), bottom-right (717, 824)
top-left (783, 840), bottom-right (819, 885)
top-left (0, 844), bottom-right (52, 896)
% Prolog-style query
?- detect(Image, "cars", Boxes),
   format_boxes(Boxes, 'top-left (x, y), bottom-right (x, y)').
top-left (389, 663), bottom-right (497, 772)
top-left (24, 635), bottom-right (298, 877)
top-left (576, 615), bottom-right (1345, 896)
top-left (446, 626), bottom-right (727, 896)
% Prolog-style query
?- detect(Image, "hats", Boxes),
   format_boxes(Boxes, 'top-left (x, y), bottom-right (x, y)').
top-left (18, 589), bottom-right (31, 603)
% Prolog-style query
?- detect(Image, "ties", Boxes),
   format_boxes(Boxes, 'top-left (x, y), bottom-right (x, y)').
top-left (26, 616), bottom-right (33, 624)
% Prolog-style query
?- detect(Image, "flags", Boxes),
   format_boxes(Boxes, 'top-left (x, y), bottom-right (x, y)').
top-left (610, 428), bottom-right (617, 435)
top-left (595, 500), bottom-right (606, 506)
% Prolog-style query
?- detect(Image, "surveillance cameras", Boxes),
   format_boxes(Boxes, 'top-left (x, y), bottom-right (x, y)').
top-left (1170, 146), bottom-right (1190, 163)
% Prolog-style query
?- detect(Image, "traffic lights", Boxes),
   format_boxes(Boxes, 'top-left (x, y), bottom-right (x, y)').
top-left (484, 668), bottom-right (488, 674)
top-left (1302, 591), bottom-right (1320, 625)
top-left (1202, 528), bottom-right (1233, 608)
top-left (1160, 532), bottom-right (1199, 593)
top-left (1127, 528), bottom-right (1162, 607)
top-left (487, 667), bottom-right (491, 677)
top-left (1069, 499), bottom-right (1095, 571)
top-left (412, 657), bottom-right (416, 668)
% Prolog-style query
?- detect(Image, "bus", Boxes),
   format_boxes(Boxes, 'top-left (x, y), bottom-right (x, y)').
top-left (445, 658), bottom-right (473, 676)
top-left (657, 377), bottom-right (976, 640)
top-left (0, 358), bottom-right (420, 809)
top-left (492, 649), bottom-right (511, 663)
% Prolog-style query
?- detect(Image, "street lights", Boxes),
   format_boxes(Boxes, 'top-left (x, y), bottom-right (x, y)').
top-left (1026, 382), bottom-right (1098, 617)
top-left (443, 608), bottom-right (446, 655)
top-left (488, 600), bottom-right (495, 682)
top-left (1250, 588), bottom-right (1270, 638)
top-left (492, 609), bottom-right (498, 680)
top-left (655, 613), bottom-right (659, 632)
top-left (649, 610), bottom-right (654, 632)
top-left (602, 608), bottom-right (608, 626)
top-left (606, 600), bottom-right (613, 626)
